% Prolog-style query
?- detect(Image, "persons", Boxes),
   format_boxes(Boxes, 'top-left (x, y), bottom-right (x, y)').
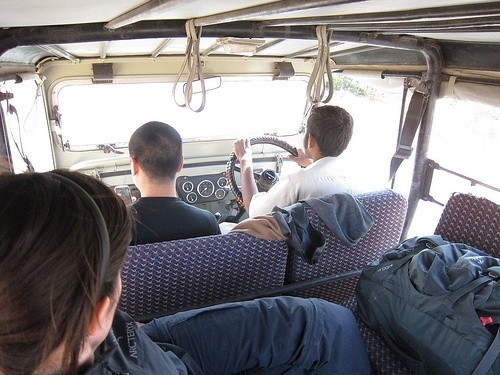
top-left (0, 169), bottom-right (189, 375)
top-left (124, 121), bottom-right (222, 247)
top-left (233, 105), bottom-right (360, 219)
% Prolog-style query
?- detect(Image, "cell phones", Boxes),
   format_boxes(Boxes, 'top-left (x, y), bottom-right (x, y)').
top-left (114, 186), bottom-right (132, 206)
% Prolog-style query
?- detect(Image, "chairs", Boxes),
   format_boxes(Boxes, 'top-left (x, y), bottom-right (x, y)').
top-left (118, 232), bottom-right (290, 323)
top-left (337, 193), bottom-right (500, 375)
top-left (290, 189), bottom-right (408, 301)
